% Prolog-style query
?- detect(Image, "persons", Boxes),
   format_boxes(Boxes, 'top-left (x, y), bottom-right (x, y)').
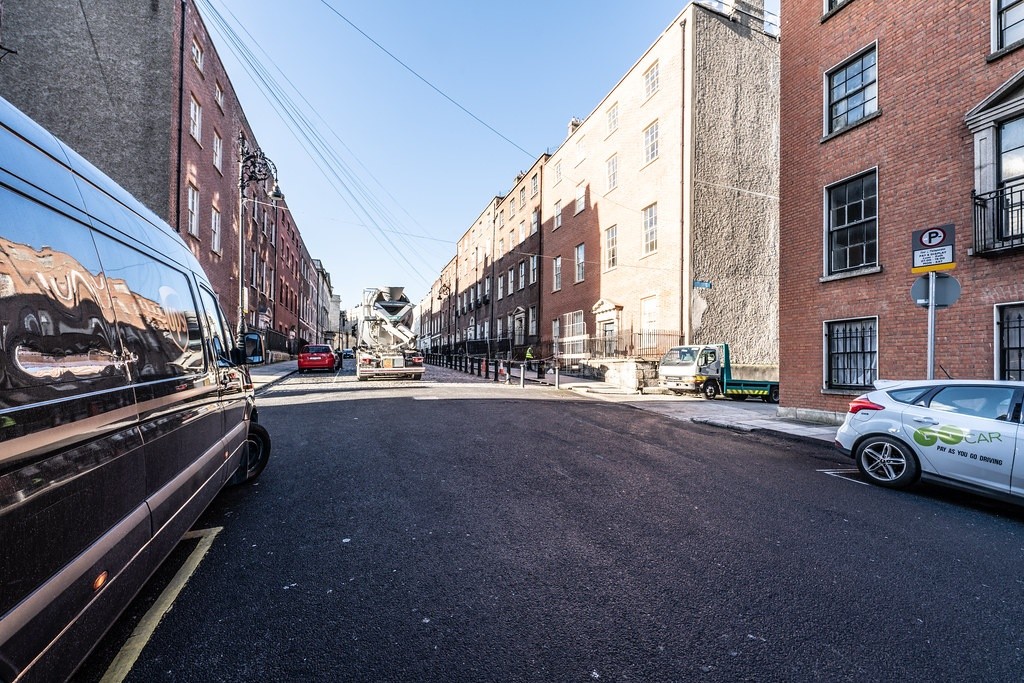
top-left (525, 342), bottom-right (537, 371)
top-left (457, 344), bottom-right (465, 362)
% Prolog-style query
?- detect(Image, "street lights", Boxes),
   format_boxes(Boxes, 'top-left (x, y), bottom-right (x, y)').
top-left (239, 130), bottom-right (285, 366)
top-left (337, 304), bottom-right (360, 369)
top-left (437, 285), bottom-right (451, 364)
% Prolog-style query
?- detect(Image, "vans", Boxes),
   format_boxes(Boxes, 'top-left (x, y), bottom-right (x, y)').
top-left (0, 93), bottom-right (272, 675)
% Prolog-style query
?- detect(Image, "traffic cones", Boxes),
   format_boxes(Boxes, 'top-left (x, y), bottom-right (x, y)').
top-left (482, 358), bottom-right (487, 372)
top-left (499, 360), bottom-right (504, 375)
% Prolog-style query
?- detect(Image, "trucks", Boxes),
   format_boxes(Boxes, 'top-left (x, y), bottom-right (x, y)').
top-left (658, 343), bottom-right (779, 403)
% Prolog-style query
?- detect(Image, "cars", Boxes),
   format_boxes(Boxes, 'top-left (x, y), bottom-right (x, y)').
top-left (297, 343), bottom-right (336, 374)
top-left (342, 348), bottom-right (354, 359)
top-left (835, 379), bottom-right (1024, 502)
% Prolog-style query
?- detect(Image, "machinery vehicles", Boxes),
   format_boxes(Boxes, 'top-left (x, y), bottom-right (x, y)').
top-left (356, 285), bottom-right (425, 381)
